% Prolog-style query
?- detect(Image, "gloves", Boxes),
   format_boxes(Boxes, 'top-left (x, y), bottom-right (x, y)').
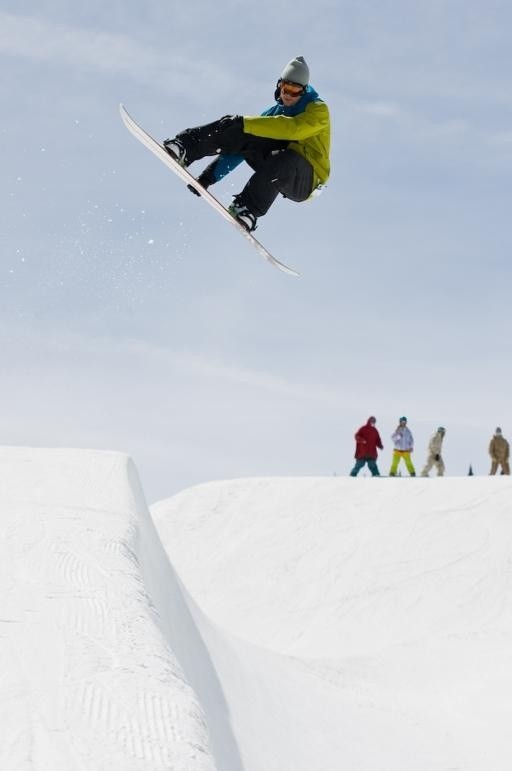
top-left (187, 171), bottom-right (217, 197)
top-left (221, 114), bottom-right (243, 132)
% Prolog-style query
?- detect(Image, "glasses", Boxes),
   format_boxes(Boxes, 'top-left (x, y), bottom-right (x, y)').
top-left (280, 87), bottom-right (301, 97)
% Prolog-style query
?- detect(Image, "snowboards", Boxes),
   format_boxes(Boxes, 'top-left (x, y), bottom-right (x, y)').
top-left (119, 102), bottom-right (302, 277)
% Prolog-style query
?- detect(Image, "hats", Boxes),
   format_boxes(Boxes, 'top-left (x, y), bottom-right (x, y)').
top-left (281, 55), bottom-right (309, 87)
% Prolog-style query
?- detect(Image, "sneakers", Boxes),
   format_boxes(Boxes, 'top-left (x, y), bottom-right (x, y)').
top-left (165, 137), bottom-right (186, 160)
top-left (230, 198), bottom-right (256, 230)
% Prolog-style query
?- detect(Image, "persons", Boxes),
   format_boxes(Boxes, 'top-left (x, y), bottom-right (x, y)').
top-left (387, 416), bottom-right (416, 477)
top-left (348, 417), bottom-right (382, 478)
top-left (419, 427), bottom-right (446, 478)
top-left (488, 428), bottom-right (510, 476)
top-left (163, 53), bottom-right (334, 230)
